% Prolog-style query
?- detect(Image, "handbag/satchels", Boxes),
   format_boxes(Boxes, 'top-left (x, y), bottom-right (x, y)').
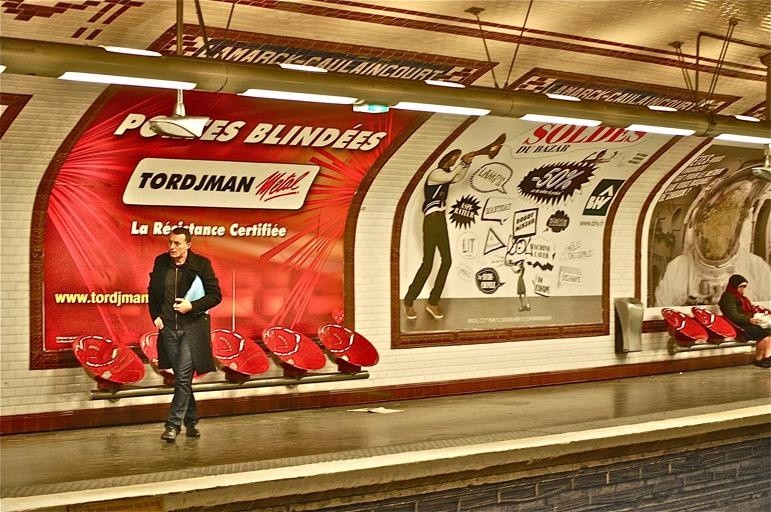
top-left (753, 312), bottom-right (771, 329)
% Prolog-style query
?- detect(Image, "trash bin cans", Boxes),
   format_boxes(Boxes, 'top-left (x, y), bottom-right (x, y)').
top-left (614, 297), bottom-right (644, 354)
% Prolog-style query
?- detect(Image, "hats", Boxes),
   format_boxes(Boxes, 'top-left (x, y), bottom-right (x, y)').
top-left (738, 282), bottom-right (747, 287)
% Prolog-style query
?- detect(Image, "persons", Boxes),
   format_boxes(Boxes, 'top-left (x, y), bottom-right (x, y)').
top-left (403, 149), bottom-right (472, 319)
top-left (653, 164), bottom-right (771, 307)
top-left (718, 275), bottom-right (771, 368)
top-left (148, 228), bottom-right (222, 442)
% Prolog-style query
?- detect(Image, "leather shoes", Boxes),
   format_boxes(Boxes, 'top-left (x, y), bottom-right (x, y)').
top-left (187, 428), bottom-right (200, 437)
top-left (161, 427), bottom-right (178, 442)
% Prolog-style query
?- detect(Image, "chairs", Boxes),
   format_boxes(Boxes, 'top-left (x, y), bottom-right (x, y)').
top-left (72, 335), bottom-right (146, 390)
top-left (661, 305), bottom-right (744, 354)
top-left (207, 328), bottom-right (270, 384)
top-left (140, 332), bottom-right (209, 386)
top-left (317, 324), bottom-right (379, 374)
top-left (261, 327), bottom-right (326, 379)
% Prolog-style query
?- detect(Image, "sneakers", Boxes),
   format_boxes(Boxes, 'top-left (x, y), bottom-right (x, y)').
top-left (753, 356), bottom-right (771, 368)
top-left (403, 300), bottom-right (418, 319)
top-left (425, 303), bottom-right (444, 319)
